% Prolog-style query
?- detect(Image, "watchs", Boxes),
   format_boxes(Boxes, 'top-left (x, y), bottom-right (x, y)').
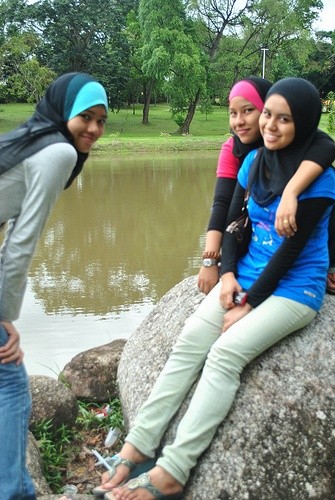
top-left (202, 259), bottom-right (218, 267)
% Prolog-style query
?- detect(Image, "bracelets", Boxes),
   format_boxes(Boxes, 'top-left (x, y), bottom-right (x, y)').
top-left (202, 251), bottom-right (220, 259)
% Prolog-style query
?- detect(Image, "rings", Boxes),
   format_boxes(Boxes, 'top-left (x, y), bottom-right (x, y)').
top-left (283, 220), bottom-right (289, 223)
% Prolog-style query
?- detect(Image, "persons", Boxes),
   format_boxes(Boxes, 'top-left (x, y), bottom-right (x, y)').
top-left (198, 78), bottom-right (335, 295)
top-left (91, 79), bottom-right (335, 499)
top-left (1, 72), bottom-right (108, 500)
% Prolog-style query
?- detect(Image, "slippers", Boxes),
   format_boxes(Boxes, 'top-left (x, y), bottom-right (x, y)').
top-left (91, 454), bottom-right (156, 496)
top-left (104, 472), bottom-right (185, 500)
